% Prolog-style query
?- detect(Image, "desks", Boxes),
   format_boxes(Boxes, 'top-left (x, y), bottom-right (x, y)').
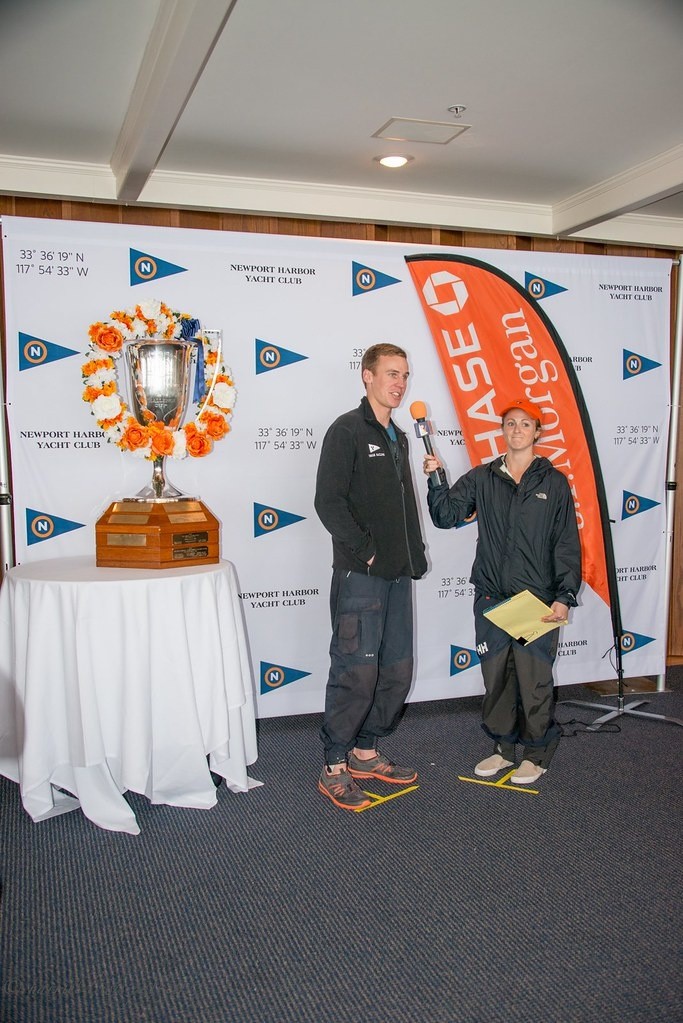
top-left (0, 554), bottom-right (265, 836)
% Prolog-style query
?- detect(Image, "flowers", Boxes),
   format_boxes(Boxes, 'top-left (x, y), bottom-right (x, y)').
top-left (81, 298), bottom-right (237, 460)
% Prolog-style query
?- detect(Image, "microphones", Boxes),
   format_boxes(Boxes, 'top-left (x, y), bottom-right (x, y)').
top-left (410, 400), bottom-right (443, 488)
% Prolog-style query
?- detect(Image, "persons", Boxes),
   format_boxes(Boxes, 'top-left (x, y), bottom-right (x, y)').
top-left (424, 399), bottom-right (583, 784)
top-left (314, 343), bottom-right (428, 809)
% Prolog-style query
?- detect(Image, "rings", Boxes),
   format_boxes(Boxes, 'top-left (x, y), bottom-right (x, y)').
top-left (426, 465), bottom-right (428, 468)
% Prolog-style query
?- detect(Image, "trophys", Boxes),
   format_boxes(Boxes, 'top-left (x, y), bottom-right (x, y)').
top-left (120, 329), bottom-right (222, 502)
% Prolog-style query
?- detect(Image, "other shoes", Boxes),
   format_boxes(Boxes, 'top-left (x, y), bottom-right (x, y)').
top-left (474, 753), bottom-right (515, 777)
top-left (510, 759), bottom-right (548, 784)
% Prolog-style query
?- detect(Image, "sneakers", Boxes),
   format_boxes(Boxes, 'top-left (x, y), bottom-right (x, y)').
top-left (347, 748), bottom-right (418, 784)
top-left (318, 763), bottom-right (373, 810)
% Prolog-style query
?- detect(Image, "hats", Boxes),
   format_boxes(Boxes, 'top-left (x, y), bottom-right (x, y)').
top-left (495, 398), bottom-right (544, 425)
